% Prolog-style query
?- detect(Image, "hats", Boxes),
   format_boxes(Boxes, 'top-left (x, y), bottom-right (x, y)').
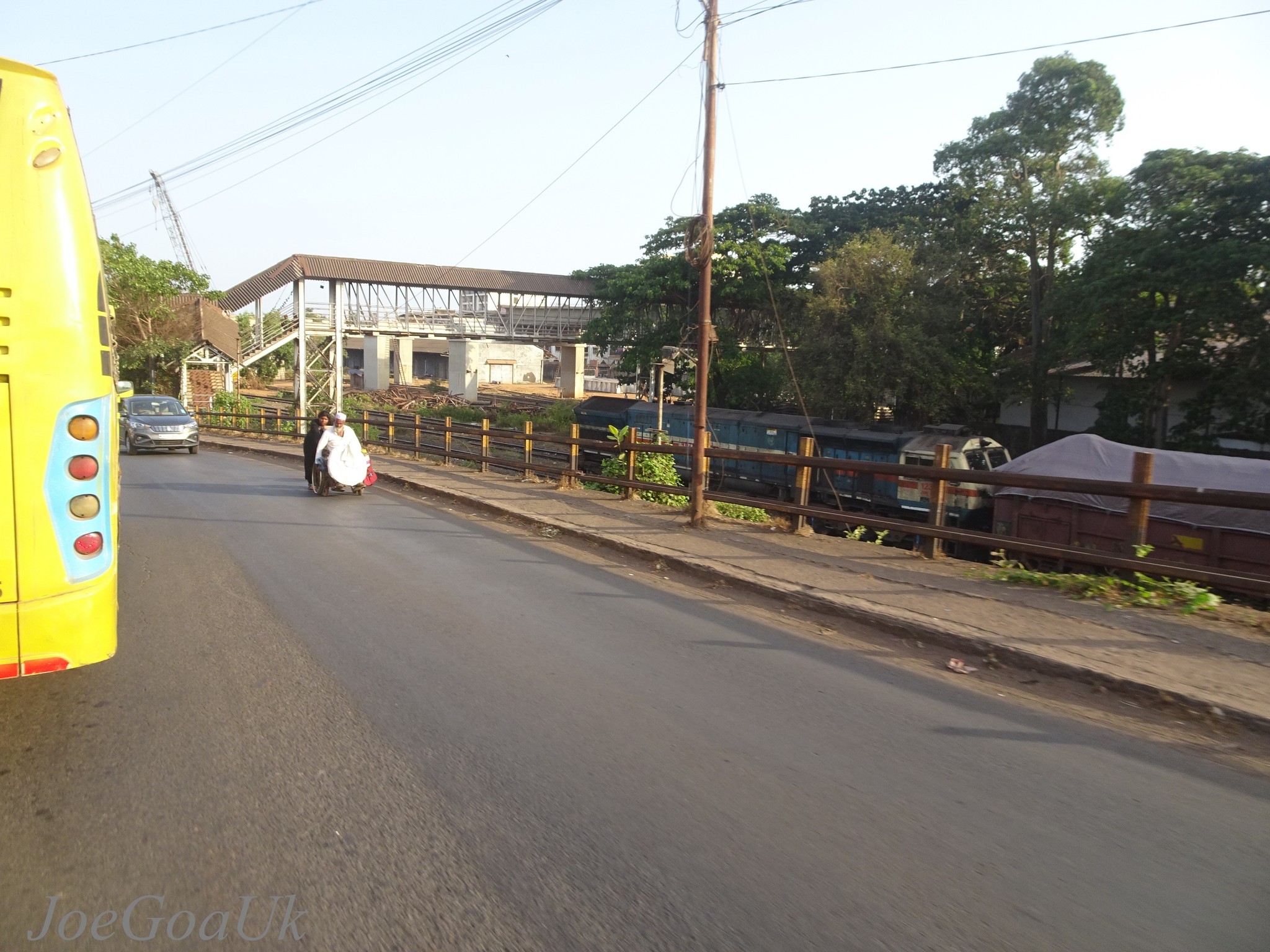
top-left (335, 412), bottom-right (347, 420)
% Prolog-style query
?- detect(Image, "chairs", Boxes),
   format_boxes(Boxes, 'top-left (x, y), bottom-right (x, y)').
top-left (158, 404), bottom-right (173, 414)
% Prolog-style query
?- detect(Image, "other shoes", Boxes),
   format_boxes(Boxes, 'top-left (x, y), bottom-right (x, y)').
top-left (336, 482), bottom-right (343, 489)
top-left (308, 483), bottom-right (316, 490)
top-left (355, 482), bottom-right (366, 488)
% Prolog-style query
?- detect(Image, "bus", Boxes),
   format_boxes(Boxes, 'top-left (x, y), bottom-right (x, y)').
top-left (0, 54), bottom-right (135, 680)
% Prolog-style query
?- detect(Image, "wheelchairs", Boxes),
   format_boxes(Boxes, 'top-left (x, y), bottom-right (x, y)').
top-left (311, 449), bottom-right (366, 495)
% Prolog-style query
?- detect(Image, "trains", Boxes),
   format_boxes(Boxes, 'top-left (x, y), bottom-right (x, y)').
top-left (569, 394), bottom-right (1269, 606)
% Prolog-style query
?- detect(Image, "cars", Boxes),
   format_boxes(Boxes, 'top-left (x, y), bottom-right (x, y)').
top-left (118, 395), bottom-right (201, 455)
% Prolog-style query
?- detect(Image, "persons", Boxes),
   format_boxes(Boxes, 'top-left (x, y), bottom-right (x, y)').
top-left (315, 413), bottom-right (367, 488)
top-left (135, 404), bottom-right (149, 415)
top-left (303, 410), bottom-right (334, 490)
